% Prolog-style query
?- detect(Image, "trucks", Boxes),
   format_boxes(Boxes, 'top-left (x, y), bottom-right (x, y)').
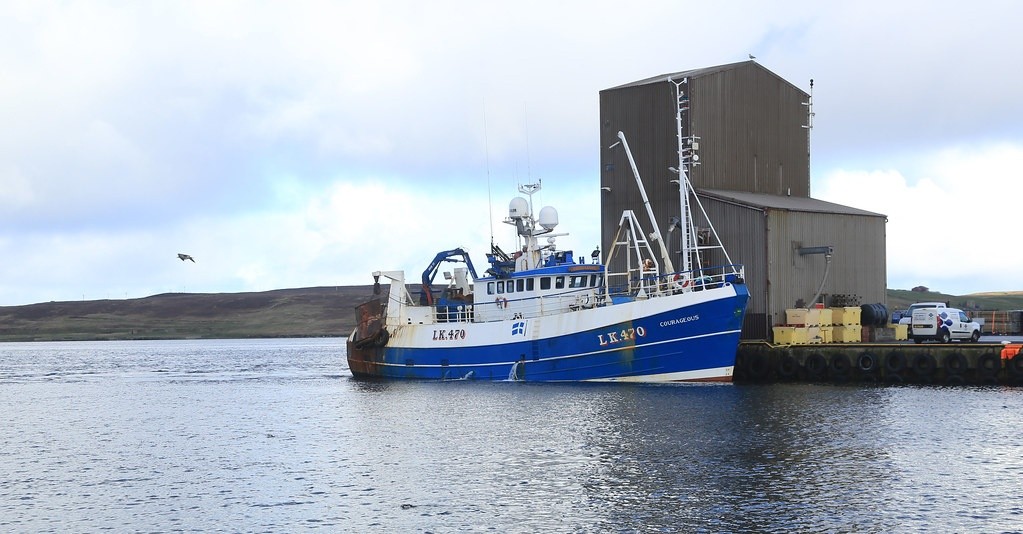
top-left (900, 302), bottom-right (985, 339)
top-left (963, 310), bottom-right (1023, 335)
top-left (890, 309), bottom-right (907, 324)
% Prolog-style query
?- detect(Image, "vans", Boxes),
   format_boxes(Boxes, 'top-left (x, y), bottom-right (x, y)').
top-left (911, 307), bottom-right (981, 343)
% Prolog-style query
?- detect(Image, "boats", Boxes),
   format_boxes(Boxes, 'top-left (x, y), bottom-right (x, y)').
top-left (346, 73), bottom-right (754, 389)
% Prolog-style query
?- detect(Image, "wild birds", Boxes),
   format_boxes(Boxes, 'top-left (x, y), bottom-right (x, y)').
top-left (748, 53), bottom-right (756, 60)
top-left (177, 253), bottom-right (196, 263)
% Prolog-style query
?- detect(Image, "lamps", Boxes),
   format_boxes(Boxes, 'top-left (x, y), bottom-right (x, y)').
top-left (555, 252), bottom-right (565, 266)
top-left (443, 271), bottom-right (452, 280)
top-left (591, 250), bottom-right (600, 264)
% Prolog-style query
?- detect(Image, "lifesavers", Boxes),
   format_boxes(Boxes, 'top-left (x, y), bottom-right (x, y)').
top-left (746, 351), bottom-right (772, 379)
top-left (780, 355), bottom-right (800, 377)
top-left (943, 351), bottom-right (968, 386)
top-left (976, 352), bottom-right (1002, 386)
top-left (804, 354), bottom-right (827, 376)
top-left (673, 273), bottom-right (689, 288)
top-left (912, 352), bottom-right (937, 378)
top-left (829, 352), bottom-right (851, 374)
top-left (856, 351), bottom-right (880, 376)
top-left (884, 351), bottom-right (908, 385)
top-left (495, 295), bottom-right (508, 310)
top-left (374, 329), bottom-right (389, 347)
top-left (1008, 352), bottom-right (1023, 386)
